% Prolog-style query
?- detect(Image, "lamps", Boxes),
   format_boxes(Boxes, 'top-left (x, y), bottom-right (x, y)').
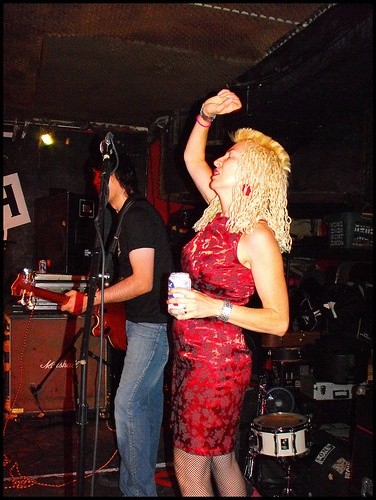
top-left (38, 124), bottom-right (56, 151)
top-left (13, 125), bottom-right (22, 143)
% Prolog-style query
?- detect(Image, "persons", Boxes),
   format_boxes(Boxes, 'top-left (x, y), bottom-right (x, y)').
top-left (61, 146), bottom-right (175, 496)
top-left (167, 89), bottom-right (292, 497)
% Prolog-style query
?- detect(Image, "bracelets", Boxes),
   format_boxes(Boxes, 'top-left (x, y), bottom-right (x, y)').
top-left (199, 109), bottom-right (213, 122)
top-left (217, 301), bottom-right (232, 322)
top-left (196, 116), bottom-right (211, 128)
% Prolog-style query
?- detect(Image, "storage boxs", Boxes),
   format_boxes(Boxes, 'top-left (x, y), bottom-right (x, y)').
top-left (325, 212), bottom-right (374, 249)
top-left (35, 192), bottom-right (100, 276)
top-left (299, 377), bottom-right (367, 400)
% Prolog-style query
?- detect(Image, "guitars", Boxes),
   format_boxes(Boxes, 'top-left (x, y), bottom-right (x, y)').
top-left (10, 273), bottom-right (128, 353)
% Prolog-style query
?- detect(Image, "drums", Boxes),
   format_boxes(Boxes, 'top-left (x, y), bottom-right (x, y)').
top-left (246, 409), bottom-right (311, 459)
top-left (269, 346), bottom-right (308, 365)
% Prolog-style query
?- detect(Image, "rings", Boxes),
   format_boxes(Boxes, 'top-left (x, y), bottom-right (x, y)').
top-left (184, 311), bottom-right (187, 313)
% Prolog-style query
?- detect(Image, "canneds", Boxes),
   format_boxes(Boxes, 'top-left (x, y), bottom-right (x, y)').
top-left (39, 260), bottom-right (47, 275)
top-left (168, 271), bottom-right (192, 317)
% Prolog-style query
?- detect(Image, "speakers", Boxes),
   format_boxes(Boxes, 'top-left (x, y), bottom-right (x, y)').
top-left (0, 304), bottom-right (111, 420)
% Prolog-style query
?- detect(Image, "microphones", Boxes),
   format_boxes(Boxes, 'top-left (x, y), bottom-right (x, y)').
top-left (100, 132), bottom-right (114, 155)
